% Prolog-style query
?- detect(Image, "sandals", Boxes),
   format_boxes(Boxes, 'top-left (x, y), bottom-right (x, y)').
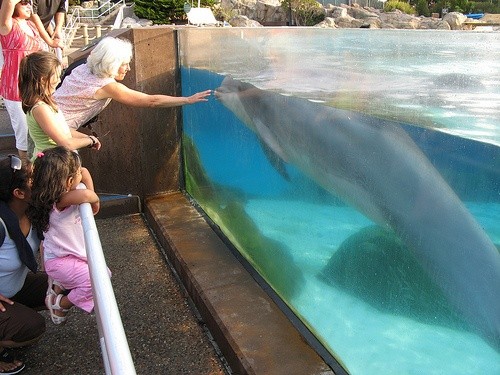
top-left (46, 279), bottom-right (66, 296)
top-left (45, 294), bottom-right (70, 325)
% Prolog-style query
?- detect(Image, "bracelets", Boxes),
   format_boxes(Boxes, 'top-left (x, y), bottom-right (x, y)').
top-left (87, 137), bottom-right (95, 148)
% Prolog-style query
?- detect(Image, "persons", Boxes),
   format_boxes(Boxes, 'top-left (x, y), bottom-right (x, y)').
top-left (0, 0), bottom-right (66, 158)
top-left (0, 156), bottom-right (72, 375)
top-left (25, 146), bottom-right (111, 325)
top-left (49, 36), bottom-right (211, 134)
top-left (18, 51), bottom-right (101, 151)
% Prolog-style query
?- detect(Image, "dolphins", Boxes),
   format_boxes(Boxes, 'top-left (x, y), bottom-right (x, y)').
top-left (213, 75), bottom-right (500, 348)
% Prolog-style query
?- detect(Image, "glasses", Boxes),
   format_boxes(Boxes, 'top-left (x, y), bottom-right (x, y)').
top-left (7, 153), bottom-right (22, 172)
top-left (19, 0), bottom-right (31, 5)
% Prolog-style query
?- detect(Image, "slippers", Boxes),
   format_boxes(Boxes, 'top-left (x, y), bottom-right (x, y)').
top-left (0, 350), bottom-right (25, 375)
